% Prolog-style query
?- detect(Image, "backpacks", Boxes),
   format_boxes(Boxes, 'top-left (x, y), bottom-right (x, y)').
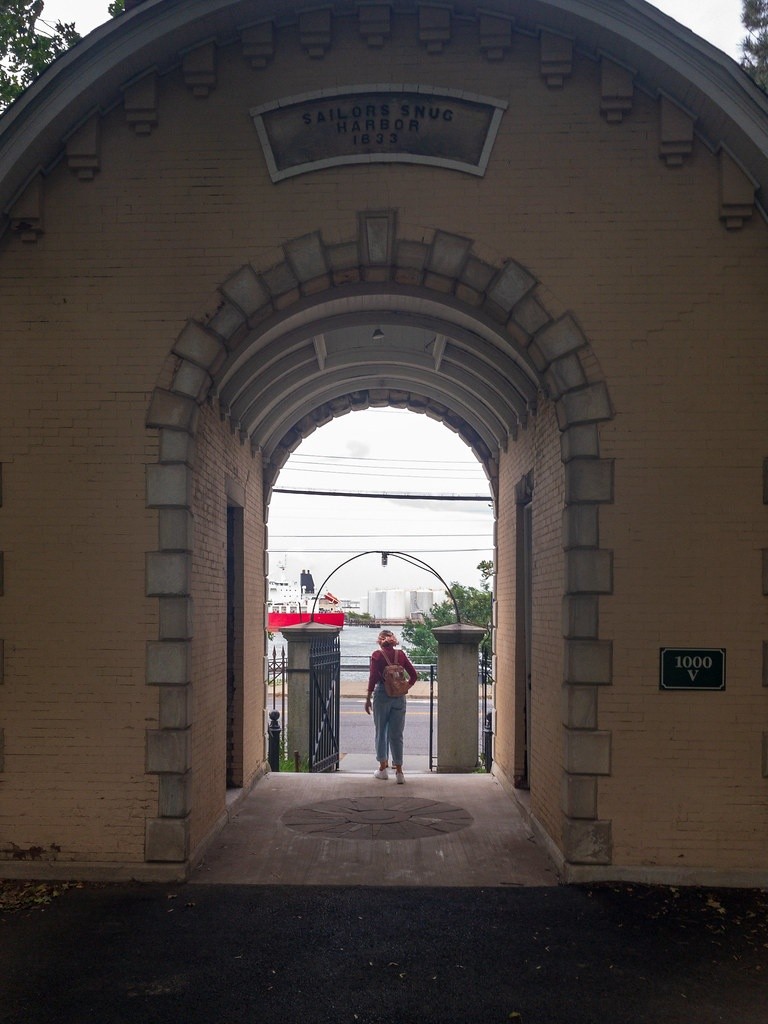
top-left (378, 649), bottom-right (409, 698)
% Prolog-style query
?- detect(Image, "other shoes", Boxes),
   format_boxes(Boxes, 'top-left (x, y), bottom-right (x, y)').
top-left (391, 760), bottom-right (397, 769)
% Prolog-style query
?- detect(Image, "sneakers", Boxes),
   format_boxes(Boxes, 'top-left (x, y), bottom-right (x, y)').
top-left (374, 766), bottom-right (388, 779)
top-left (395, 771), bottom-right (405, 784)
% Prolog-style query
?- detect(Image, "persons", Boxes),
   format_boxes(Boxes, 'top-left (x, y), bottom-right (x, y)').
top-left (364, 630), bottom-right (418, 784)
